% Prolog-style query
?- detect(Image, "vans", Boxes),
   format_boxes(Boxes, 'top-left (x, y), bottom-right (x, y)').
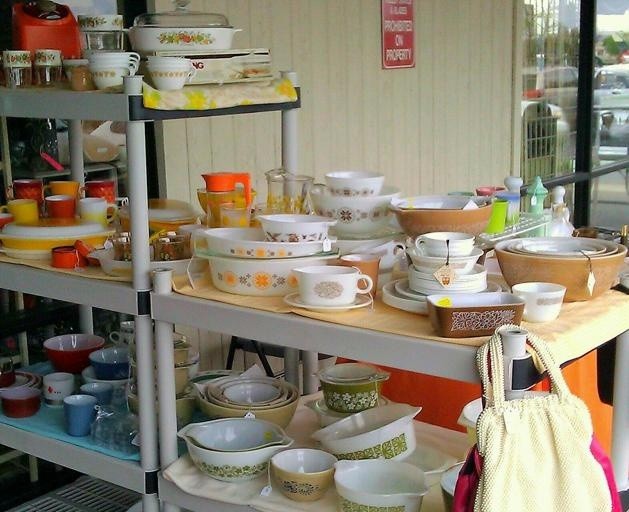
top-left (521, 66), bottom-right (578, 130)
top-left (593, 64), bottom-right (629, 108)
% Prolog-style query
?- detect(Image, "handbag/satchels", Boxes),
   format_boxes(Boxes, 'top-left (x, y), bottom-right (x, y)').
top-left (473, 324), bottom-right (609, 512)
top-left (452, 338), bottom-right (621, 511)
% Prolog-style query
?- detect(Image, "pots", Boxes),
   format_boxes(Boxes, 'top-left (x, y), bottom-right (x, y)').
top-left (0, 224), bottom-right (116, 251)
top-left (124, 1), bottom-right (244, 53)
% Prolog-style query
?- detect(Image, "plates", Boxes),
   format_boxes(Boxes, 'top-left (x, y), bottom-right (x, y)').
top-left (0, 370), bottom-right (34, 390)
top-left (394, 278), bottom-right (428, 302)
top-left (0, 247), bottom-right (51, 261)
top-left (25, 372), bottom-right (41, 390)
top-left (381, 276), bottom-right (502, 315)
top-left (282, 292), bottom-right (373, 313)
top-left (94, 250), bottom-right (204, 278)
top-left (407, 264), bottom-right (489, 296)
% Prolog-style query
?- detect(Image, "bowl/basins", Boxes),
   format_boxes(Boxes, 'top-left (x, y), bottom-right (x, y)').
top-left (255, 214), bottom-right (338, 242)
top-left (405, 247), bottom-right (486, 275)
top-left (514, 243), bottom-right (608, 255)
top-left (305, 395), bottom-right (394, 430)
top-left (80, 366), bottom-right (138, 391)
top-left (0, 387), bottom-right (42, 418)
top-left (461, 397), bottom-right (482, 446)
top-left (194, 252), bottom-right (341, 298)
top-left (310, 403), bottom-right (422, 463)
top-left (204, 226), bottom-right (340, 256)
top-left (492, 236), bottom-right (628, 303)
top-left (223, 383), bottom-right (282, 404)
top-left (118, 198), bottom-right (198, 231)
top-left (132, 344), bottom-right (193, 364)
top-left (311, 362), bottom-right (390, 413)
top-left (324, 362), bottom-right (378, 382)
top-left (520, 245), bottom-right (598, 252)
top-left (506, 238), bottom-right (620, 258)
top-left (176, 416), bottom-right (295, 484)
top-left (426, 291), bottom-right (526, 338)
top-left (440, 461), bottom-right (466, 512)
top-left (184, 419), bottom-right (288, 453)
top-left (333, 458), bottom-right (430, 512)
top-left (126, 380), bottom-right (200, 427)
top-left (269, 448), bottom-right (338, 502)
top-left (193, 375), bottom-right (301, 431)
top-left (309, 185), bottom-right (401, 233)
top-left (217, 378), bottom-right (290, 408)
top-left (43, 333), bottom-right (105, 374)
top-left (130, 358), bottom-right (200, 397)
top-left (387, 195), bottom-right (494, 241)
top-left (325, 170), bottom-right (386, 197)
top-left (207, 376), bottom-right (297, 411)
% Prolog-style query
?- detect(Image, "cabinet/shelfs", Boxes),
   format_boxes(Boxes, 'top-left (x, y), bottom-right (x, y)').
top-left (150, 266), bottom-right (628, 511)
top-left (0, 72), bottom-right (301, 511)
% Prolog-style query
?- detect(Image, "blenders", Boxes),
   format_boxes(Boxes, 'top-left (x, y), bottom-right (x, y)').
top-left (195, 170), bottom-right (256, 220)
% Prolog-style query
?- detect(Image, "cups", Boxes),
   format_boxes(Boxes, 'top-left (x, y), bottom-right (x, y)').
top-left (108, 237), bottom-right (133, 263)
top-left (160, 231), bottom-right (192, 258)
top-left (34, 49), bottom-right (63, 88)
top-left (218, 201), bottom-right (252, 228)
top-left (0, 370), bottom-right (16, 387)
top-left (77, 14), bottom-right (129, 51)
top-left (494, 190), bottom-right (521, 226)
top-left (152, 237), bottom-right (186, 262)
top-left (48, 180), bottom-right (80, 198)
top-left (80, 381), bottom-right (114, 405)
top-left (338, 252), bottom-right (381, 300)
top-left (0, 198), bottom-right (40, 225)
top-left (351, 239), bottom-right (407, 269)
top-left (88, 50), bottom-right (141, 91)
top-left (145, 55), bottom-right (196, 92)
top-left (79, 179), bottom-right (116, 215)
top-left (2, 49), bottom-right (33, 89)
top-left (0, 355), bottom-right (13, 371)
top-left (484, 200), bottom-right (509, 235)
top-left (119, 320), bottom-right (135, 333)
top-left (108, 332), bottom-right (136, 349)
top-left (43, 372), bottom-right (75, 409)
top-left (112, 231), bottom-right (133, 238)
top-left (475, 187), bottom-right (505, 196)
top-left (79, 197), bottom-right (118, 226)
top-left (89, 346), bottom-right (133, 380)
top-left (414, 231), bottom-right (476, 257)
top-left (62, 394), bottom-right (99, 438)
top-left (13, 177), bottom-right (43, 219)
top-left (51, 245), bottom-right (87, 269)
top-left (511, 281), bottom-right (567, 323)
top-left (264, 167), bottom-right (316, 214)
top-left (290, 265), bottom-right (374, 306)
top-left (45, 193), bottom-right (76, 219)
